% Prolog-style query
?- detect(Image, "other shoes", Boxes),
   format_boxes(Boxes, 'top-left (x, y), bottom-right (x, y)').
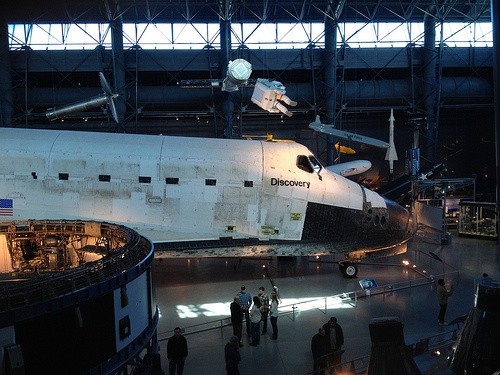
top-left (240, 331), bottom-right (278, 347)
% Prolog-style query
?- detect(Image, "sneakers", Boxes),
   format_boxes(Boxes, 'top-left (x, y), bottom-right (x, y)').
top-left (437, 319), bottom-right (449, 327)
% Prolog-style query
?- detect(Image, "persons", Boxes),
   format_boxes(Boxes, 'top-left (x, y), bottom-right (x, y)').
top-left (250, 297), bottom-right (261, 346)
top-left (258, 287), bottom-right (278, 339)
top-left (230, 285), bottom-right (252, 347)
top-left (224, 336), bottom-right (241, 375)
top-left (437, 279), bottom-right (454, 326)
top-left (311, 317), bottom-right (344, 375)
top-left (481, 272), bottom-right (492, 280)
top-left (167, 327), bottom-right (188, 375)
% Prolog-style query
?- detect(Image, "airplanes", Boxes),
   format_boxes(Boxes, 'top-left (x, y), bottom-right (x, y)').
top-left (0, 128), bottom-right (418, 277)
